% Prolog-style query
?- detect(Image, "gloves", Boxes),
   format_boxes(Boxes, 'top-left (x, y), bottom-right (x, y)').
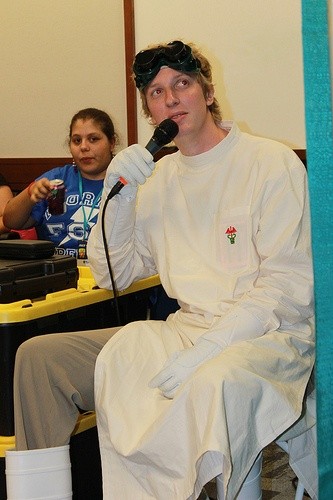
top-left (88, 143), bottom-right (156, 247)
top-left (148, 301), bottom-right (281, 400)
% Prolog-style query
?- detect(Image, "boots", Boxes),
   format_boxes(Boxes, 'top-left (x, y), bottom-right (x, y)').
top-left (5, 445), bottom-right (72, 500)
top-left (216, 451), bottom-right (263, 500)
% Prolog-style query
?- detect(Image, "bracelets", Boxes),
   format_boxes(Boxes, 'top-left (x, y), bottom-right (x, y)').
top-left (28, 181), bottom-right (35, 196)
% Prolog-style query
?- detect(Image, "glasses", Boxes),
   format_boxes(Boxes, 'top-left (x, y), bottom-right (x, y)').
top-left (133, 41), bottom-right (202, 90)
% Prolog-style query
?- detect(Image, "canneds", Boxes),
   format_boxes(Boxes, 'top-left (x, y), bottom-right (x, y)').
top-left (47, 179), bottom-right (66, 216)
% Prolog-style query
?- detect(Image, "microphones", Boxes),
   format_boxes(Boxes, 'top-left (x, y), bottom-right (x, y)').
top-left (107, 119), bottom-right (179, 199)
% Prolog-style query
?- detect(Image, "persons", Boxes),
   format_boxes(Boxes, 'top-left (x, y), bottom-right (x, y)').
top-left (5, 39), bottom-right (317, 500)
top-left (9, 107), bottom-right (116, 266)
top-left (0, 171), bottom-right (16, 232)
